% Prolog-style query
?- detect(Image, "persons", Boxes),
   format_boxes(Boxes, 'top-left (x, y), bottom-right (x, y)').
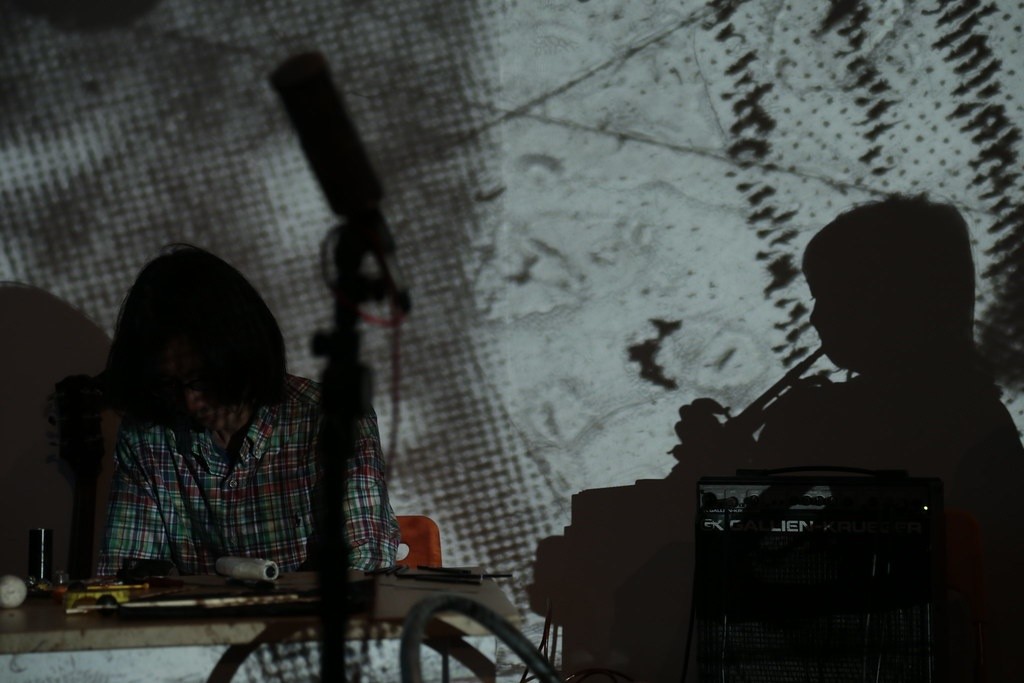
top-left (99, 246), bottom-right (402, 583)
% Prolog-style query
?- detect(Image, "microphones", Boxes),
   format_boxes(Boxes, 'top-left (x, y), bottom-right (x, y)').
top-left (270, 52), bottom-right (410, 315)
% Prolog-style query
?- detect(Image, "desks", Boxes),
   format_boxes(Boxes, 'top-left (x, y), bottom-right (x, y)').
top-left (0, 566), bottom-right (522, 683)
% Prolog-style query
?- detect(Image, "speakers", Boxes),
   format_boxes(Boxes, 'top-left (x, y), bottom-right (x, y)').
top-left (694, 466), bottom-right (945, 683)
